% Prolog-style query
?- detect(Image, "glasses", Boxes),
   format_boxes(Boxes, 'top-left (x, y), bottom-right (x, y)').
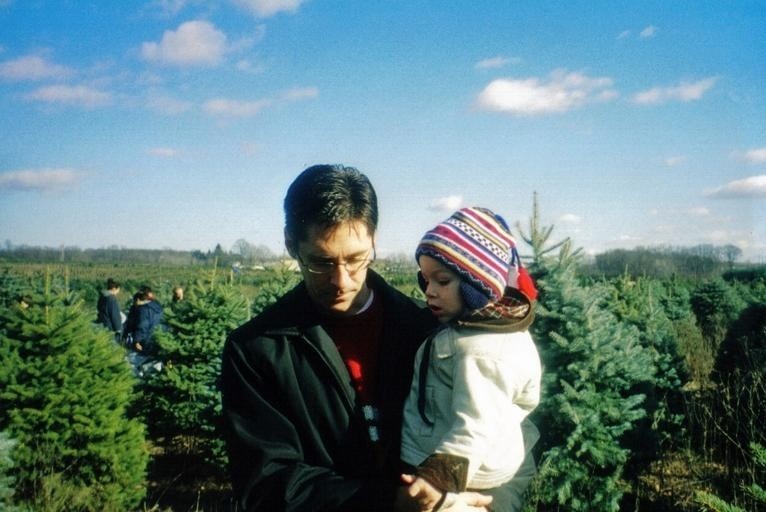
top-left (288, 240), bottom-right (377, 274)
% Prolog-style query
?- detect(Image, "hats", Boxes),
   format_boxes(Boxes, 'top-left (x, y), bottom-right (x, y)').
top-left (415, 205), bottom-right (515, 300)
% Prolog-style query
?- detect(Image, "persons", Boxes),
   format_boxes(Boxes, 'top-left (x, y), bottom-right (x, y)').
top-left (222, 163), bottom-right (539, 512)
top-left (97, 277), bottom-right (121, 345)
top-left (131, 292), bottom-right (168, 378)
top-left (397, 206), bottom-right (543, 512)
top-left (122, 285), bottom-right (160, 352)
top-left (172, 286), bottom-right (183, 302)
